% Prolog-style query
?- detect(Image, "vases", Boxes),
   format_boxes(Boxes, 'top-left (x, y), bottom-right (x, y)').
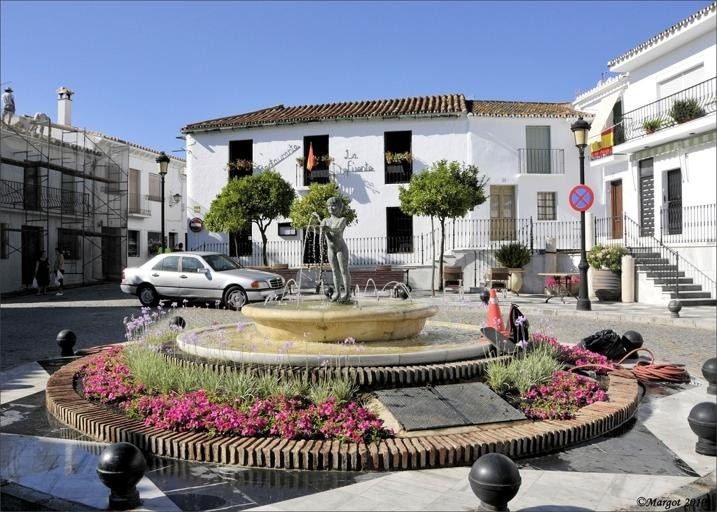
top-left (545, 286), bottom-right (569, 297)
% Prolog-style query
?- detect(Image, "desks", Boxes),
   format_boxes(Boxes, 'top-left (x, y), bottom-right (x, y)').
top-left (539, 272), bottom-right (580, 304)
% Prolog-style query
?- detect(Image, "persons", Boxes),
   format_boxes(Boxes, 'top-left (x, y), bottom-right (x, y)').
top-left (157, 236), bottom-right (171, 254)
top-left (173, 243), bottom-right (184, 252)
top-left (0, 87), bottom-right (15, 131)
top-left (52, 248), bottom-right (64, 296)
top-left (305, 197), bottom-right (351, 303)
top-left (34, 250), bottom-right (51, 296)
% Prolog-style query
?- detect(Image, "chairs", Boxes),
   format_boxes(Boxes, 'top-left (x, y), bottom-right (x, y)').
top-left (485, 268), bottom-right (508, 298)
top-left (443, 266), bottom-right (464, 301)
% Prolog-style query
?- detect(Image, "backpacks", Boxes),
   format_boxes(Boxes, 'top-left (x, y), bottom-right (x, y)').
top-left (505, 303), bottom-right (529, 342)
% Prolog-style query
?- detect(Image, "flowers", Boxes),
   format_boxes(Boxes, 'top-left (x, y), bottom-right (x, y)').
top-left (545, 277), bottom-right (579, 288)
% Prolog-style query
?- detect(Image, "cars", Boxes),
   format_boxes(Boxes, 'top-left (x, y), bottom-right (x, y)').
top-left (119, 251), bottom-right (289, 311)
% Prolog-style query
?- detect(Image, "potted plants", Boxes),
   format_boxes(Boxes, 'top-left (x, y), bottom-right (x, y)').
top-left (643, 119), bottom-right (661, 134)
top-left (668, 98), bottom-right (707, 124)
top-left (587, 242), bottom-right (627, 303)
top-left (495, 242), bottom-right (534, 293)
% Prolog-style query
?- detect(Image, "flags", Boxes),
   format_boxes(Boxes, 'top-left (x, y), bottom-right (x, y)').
top-left (589, 127), bottom-right (613, 158)
top-left (306, 142), bottom-right (316, 171)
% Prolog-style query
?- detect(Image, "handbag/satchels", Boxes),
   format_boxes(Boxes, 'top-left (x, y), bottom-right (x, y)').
top-left (577, 330), bottom-right (625, 360)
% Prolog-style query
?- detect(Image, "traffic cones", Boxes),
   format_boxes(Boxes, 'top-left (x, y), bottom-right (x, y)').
top-left (479, 288), bottom-right (509, 341)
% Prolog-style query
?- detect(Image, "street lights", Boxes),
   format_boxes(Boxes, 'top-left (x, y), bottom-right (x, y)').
top-left (155, 150), bottom-right (170, 253)
top-left (570, 117), bottom-right (591, 310)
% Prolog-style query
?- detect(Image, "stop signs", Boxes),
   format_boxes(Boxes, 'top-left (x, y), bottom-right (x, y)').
top-left (189, 218), bottom-right (204, 233)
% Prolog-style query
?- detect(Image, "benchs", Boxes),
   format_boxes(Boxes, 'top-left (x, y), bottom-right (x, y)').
top-left (262, 269), bottom-right (298, 294)
top-left (320, 270), bottom-right (411, 299)
